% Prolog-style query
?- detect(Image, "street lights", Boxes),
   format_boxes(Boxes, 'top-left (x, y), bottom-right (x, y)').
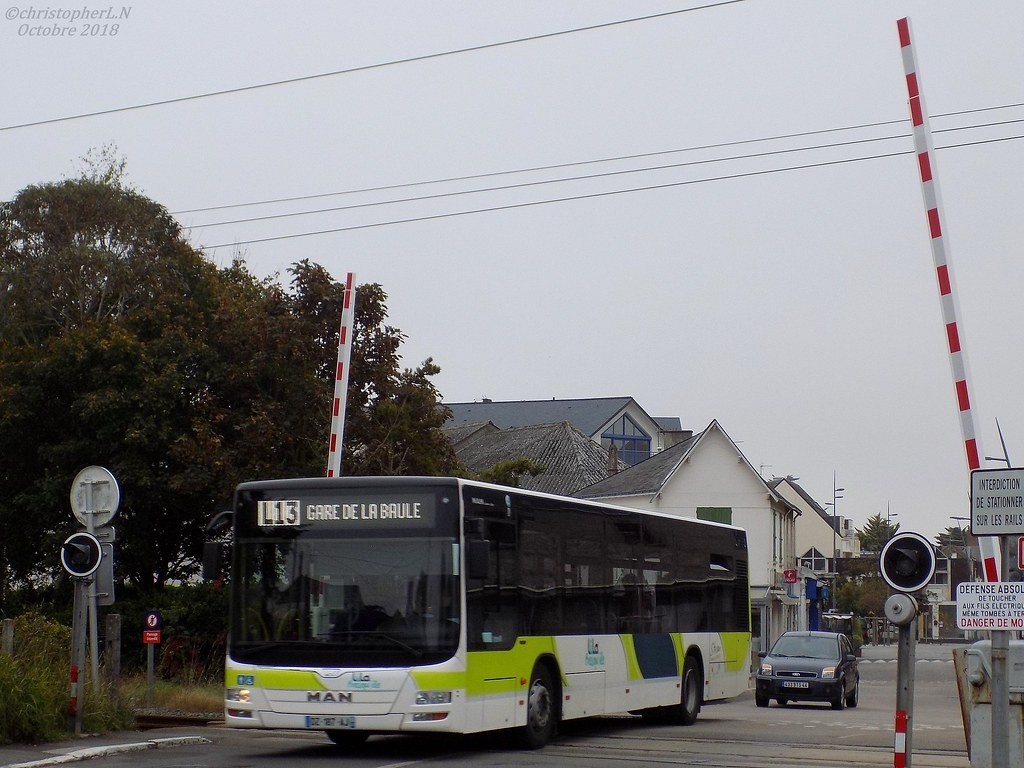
top-left (883, 500), bottom-right (898, 540)
top-left (824, 469), bottom-right (844, 609)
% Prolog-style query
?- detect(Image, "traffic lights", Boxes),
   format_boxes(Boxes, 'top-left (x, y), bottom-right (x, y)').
top-left (62, 531), bottom-right (103, 578)
top-left (879, 531), bottom-right (935, 592)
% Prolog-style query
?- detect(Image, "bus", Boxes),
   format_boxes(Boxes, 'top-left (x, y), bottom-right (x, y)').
top-left (224, 475), bottom-right (753, 751)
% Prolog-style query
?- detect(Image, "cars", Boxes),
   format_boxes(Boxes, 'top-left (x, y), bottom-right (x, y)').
top-left (755, 631), bottom-right (860, 710)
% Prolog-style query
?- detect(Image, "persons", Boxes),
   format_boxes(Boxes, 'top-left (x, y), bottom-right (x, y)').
top-left (864, 628), bottom-right (870, 646)
top-left (329, 594), bottom-right (391, 643)
